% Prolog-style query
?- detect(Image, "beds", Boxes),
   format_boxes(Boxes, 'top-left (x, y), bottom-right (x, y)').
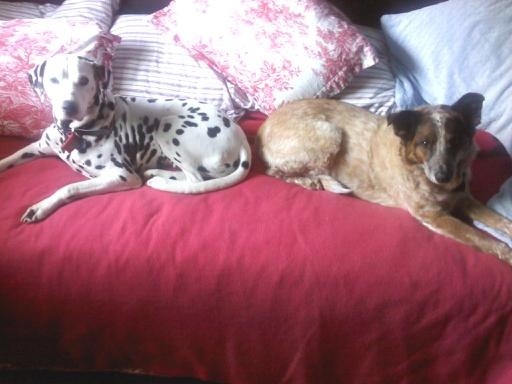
top-left (0, 129), bottom-right (511, 384)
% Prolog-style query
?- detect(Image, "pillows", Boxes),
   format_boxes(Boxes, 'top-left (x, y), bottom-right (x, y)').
top-left (379, 2), bottom-right (512, 221)
top-left (0, 16), bottom-right (121, 150)
top-left (335, 23), bottom-right (397, 114)
top-left (104, 12), bottom-right (246, 121)
top-left (54, 1), bottom-right (119, 34)
top-left (148, 1), bottom-right (378, 118)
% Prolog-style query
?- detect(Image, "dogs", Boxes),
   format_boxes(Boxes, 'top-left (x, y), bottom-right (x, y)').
top-left (0, 48), bottom-right (253, 224)
top-left (251, 92), bottom-right (512, 268)
